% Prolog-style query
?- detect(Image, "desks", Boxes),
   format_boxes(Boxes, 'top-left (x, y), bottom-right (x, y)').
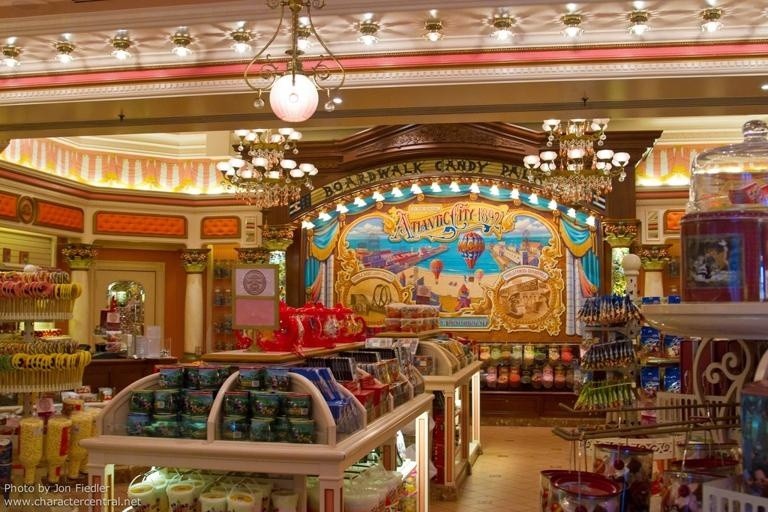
top-left (639, 301), bottom-right (768, 459)
top-left (83, 358), bottom-right (178, 400)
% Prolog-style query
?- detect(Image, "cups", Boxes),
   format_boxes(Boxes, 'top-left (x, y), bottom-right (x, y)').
top-left (738, 378), bottom-right (767, 494)
top-left (145, 326), bottom-right (162, 358)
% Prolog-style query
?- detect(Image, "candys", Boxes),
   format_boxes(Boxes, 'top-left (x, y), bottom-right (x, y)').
top-left (0, 337), bottom-right (93, 389)
top-left (0, 268), bottom-right (84, 316)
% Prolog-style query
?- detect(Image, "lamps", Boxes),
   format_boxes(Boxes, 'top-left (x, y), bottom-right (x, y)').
top-left (523, 117), bottom-right (631, 207)
top-left (300, 174), bottom-right (596, 231)
top-left (244, 0), bottom-right (346, 124)
top-left (217, 128), bottom-right (320, 210)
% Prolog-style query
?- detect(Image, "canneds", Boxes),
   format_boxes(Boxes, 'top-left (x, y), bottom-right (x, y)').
top-left (0, 438), bottom-right (12, 490)
top-left (125, 364), bottom-right (319, 445)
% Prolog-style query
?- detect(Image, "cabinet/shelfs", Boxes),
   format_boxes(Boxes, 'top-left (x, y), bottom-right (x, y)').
top-left (365, 328), bottom-right (484, 502)
top-left (76, 343), bottom-right (435, 512)
top-left (0, 313), bottom-right (105, 394)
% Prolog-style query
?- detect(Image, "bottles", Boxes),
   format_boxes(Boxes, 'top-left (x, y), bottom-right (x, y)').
top-left (19, 407), bottom-right (102, 488)
top-left (121, 334), bottom-right (145, 359)
